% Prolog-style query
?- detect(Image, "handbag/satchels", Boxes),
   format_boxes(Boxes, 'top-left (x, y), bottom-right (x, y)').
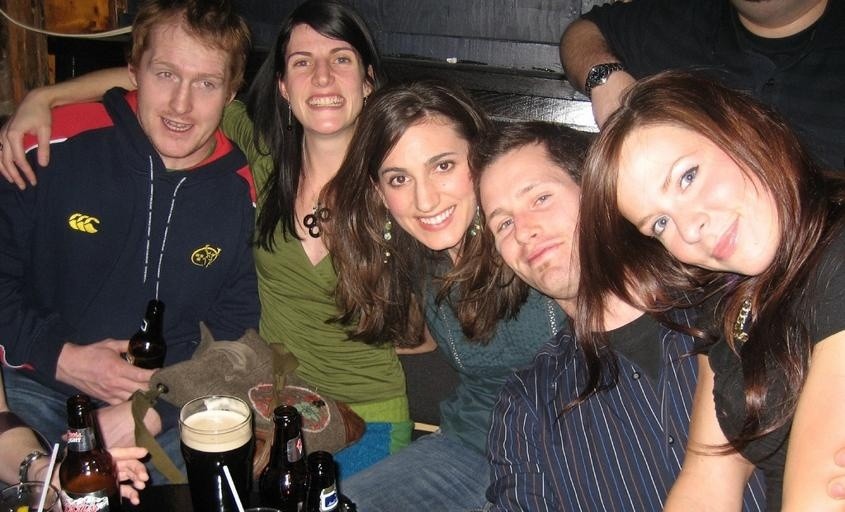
top-left (129, 319), bottom-right (366, 500)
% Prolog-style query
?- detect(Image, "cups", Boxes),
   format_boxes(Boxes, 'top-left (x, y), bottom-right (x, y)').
top-left (0, 481), bottom-right (63, 512)
top-left (178, 392), bottom-right (257, 511)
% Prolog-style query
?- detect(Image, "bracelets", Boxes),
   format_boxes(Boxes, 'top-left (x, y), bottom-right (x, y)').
top-left (17, 449), bottom-right (49, 500)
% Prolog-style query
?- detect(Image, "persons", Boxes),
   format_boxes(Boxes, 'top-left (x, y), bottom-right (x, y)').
top-left (568, 68), bottom-right (845, 510)
top-left (0, 1), bottom-right (262, 483)
top-left (558, 0), bottom-right (844, 182)
top-left (0, 362), bottom-right (150, 511)
top-left (467, 117), bottom-right (771, 511)
top-left (0, 1), bottom-right (413, 491)
top-left (316, 78), bottom-right (571, 510)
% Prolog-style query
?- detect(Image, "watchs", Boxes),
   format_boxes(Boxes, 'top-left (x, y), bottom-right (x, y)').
top-left (585, 62), bottom-right (626, 102)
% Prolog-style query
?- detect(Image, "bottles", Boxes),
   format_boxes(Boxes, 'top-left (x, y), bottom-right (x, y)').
top-left (59, 392), bottom-right (122, 511)
top-left (126, 297), bottom-right (169, 370)
top-left (304, 450), bottom-right (358, 511)
top-left (255, 405), bottom-right (309, 512)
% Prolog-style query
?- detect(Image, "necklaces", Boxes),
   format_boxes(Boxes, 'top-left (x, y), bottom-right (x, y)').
top-left (303, 149), bottom-right (332, 238)
top-left (732, 297), bottom-right (752, 343)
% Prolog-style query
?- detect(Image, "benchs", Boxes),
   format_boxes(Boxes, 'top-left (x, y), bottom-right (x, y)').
top-left (44, 32), bottom-right (602, 144)
